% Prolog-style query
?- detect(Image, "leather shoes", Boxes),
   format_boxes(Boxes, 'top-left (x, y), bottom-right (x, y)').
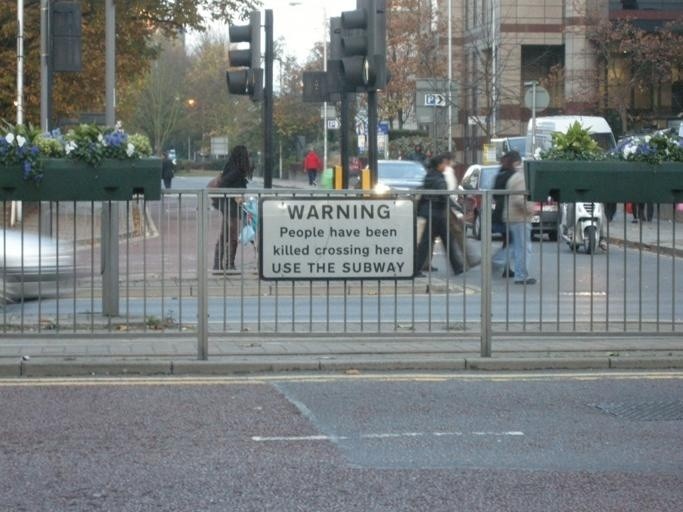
top-left (502, 268), bottom-right (536, 284)
top-left (412, 262), bottom-right (439, 279)
top-left (456, 257), bottom-right (482, 276)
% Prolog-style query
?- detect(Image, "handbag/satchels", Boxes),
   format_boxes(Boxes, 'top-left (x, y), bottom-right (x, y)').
top-left (417, 198), bottom-right (446, 220)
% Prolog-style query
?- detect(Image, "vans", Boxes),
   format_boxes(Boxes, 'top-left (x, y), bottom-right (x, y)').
top-left (525, 115), bottom-right (618, 154)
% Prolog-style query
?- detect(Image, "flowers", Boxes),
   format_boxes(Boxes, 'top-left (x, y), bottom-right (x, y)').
top-left (612, 128), bottom-right (683, 173)
top-left (0, 119), bottom-right (153, 189)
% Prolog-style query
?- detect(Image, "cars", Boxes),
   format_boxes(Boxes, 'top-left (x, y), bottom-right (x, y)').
top-left (354, 159), bottom-right (427, 198)
top-left (458, 163), bottom-right (558, 239)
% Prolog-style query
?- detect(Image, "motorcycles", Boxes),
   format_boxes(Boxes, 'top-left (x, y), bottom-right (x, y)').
top-left (560, 201), bottom-right (603, 254)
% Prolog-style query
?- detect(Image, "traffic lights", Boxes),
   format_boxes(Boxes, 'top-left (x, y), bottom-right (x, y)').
top-left (227, 10), bottom-right (265, 102)
top-left (337, 2), bottom-right (390, 92)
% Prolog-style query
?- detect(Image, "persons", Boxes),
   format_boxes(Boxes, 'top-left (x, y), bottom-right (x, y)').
top-left (492, 153), bottom-right (537, 284)
top-left (212, 146), bottom-right (253, 275)
top-left (304, 148), bottom-right (323, 186)
top-left (415, 153), bottom-right (481, 275)
top-left (631, 203), bottom-right (646, 222)
top-left (160, 153), bottom-right (176, 188)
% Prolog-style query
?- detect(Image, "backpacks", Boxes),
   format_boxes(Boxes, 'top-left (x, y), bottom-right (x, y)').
top-left (206, 170), bottom-right (233, 212)
